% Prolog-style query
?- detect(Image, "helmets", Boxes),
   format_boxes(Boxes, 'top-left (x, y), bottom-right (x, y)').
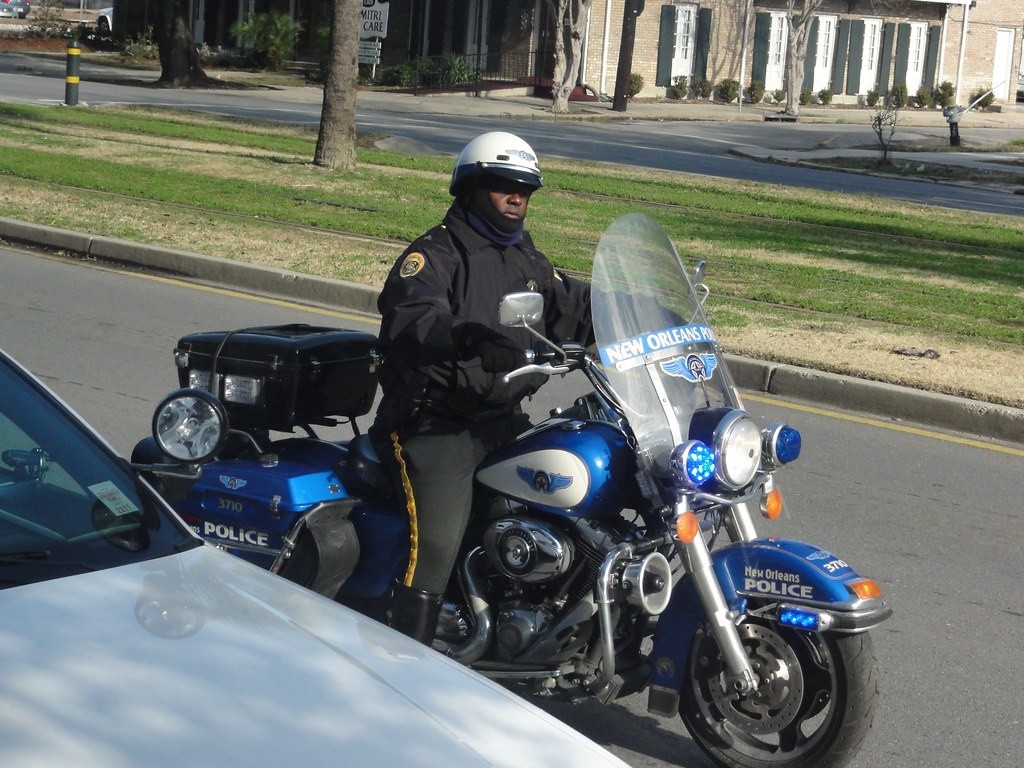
top-left (449, 131), bottom-right (543, 196)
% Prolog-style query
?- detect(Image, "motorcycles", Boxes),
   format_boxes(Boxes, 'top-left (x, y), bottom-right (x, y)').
top-left (131, 214), bottom-right (894, 768)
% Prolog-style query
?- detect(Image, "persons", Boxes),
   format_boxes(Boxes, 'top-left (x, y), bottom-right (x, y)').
top-left (367, 130), bottom-right (711, 648)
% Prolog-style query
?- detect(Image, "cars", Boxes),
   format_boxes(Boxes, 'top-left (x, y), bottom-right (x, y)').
top-left (0, 348), bottom-right (632, 768)
top-left (0, 0), bottom-right (31, 18)
top-left (96, 7), bottom-right (113, 31)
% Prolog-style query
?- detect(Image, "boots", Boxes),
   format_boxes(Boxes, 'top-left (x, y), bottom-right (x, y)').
top-left (390, 578), bottom-right (446, 647)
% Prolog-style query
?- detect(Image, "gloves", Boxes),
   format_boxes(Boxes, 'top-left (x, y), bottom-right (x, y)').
top-left (652, 307), bottom-right (688, 331)
top-left (456, 322), bottom-right (527, 374)
